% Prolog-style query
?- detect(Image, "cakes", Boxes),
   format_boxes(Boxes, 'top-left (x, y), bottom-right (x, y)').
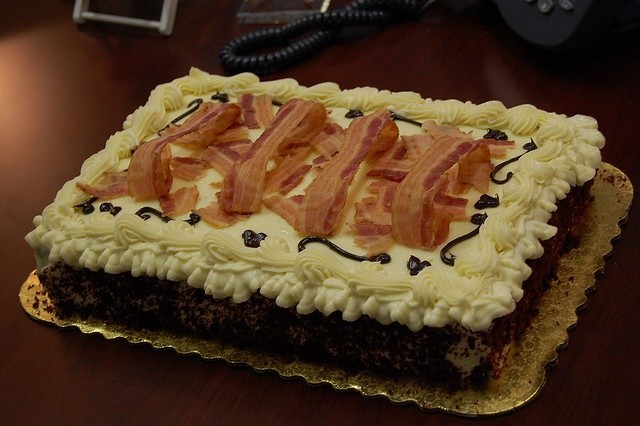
top-left (23, 65), bottom-right (606, 390)
